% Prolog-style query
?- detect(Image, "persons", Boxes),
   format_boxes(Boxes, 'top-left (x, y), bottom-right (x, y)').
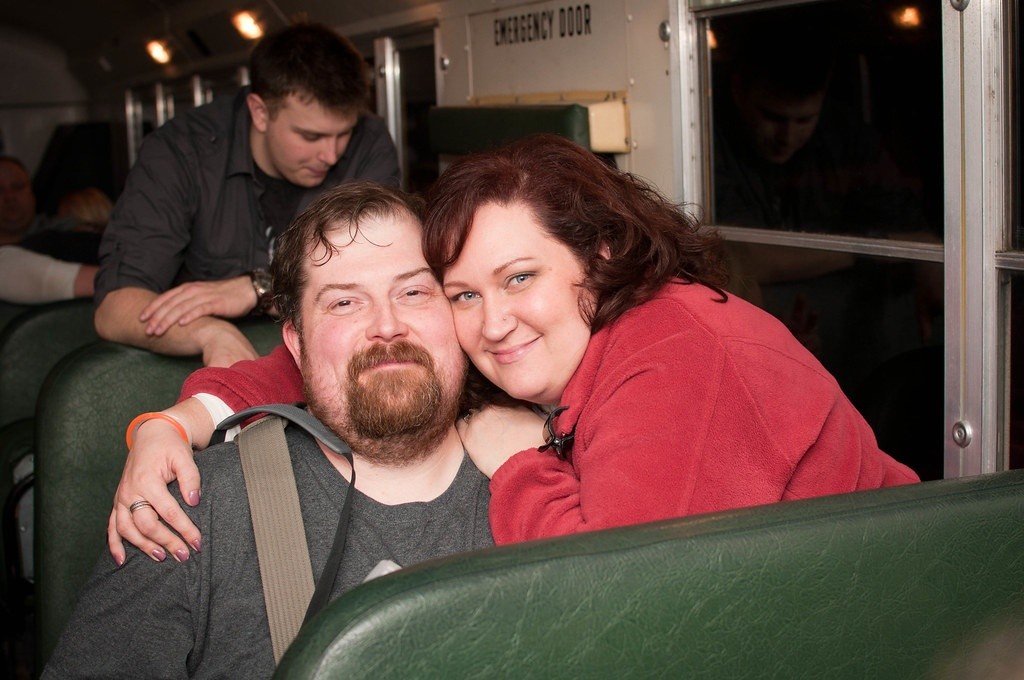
top-left (421, 133), bottom-right (918, 545)
top-left (36, 181), bottom-right (491, 680)
top-left (0, 154), bottom-right (113, 304)
top-left (91, 21), bottom-right (401, 367)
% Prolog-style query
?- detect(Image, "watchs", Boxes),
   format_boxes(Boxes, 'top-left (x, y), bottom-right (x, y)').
top-left (237, 271), bottom-right (273, 317)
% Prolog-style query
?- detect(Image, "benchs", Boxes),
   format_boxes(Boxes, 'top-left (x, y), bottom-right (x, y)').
top-left (271, 466), bottom-right (1024, 680)
top-left (37, 320), bottom-right (284, 666)
top-left (0, 299), bottom-right (98, 429)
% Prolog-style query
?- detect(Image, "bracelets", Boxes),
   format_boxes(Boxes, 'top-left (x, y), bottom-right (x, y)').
top-left (126, 412), bottom-right (191, 452)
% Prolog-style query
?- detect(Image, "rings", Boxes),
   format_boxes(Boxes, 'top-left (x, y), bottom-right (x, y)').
top-left (130, 500), bottom-right (152, 511)
top-left (462, 412), bottom-right (470, 421)
